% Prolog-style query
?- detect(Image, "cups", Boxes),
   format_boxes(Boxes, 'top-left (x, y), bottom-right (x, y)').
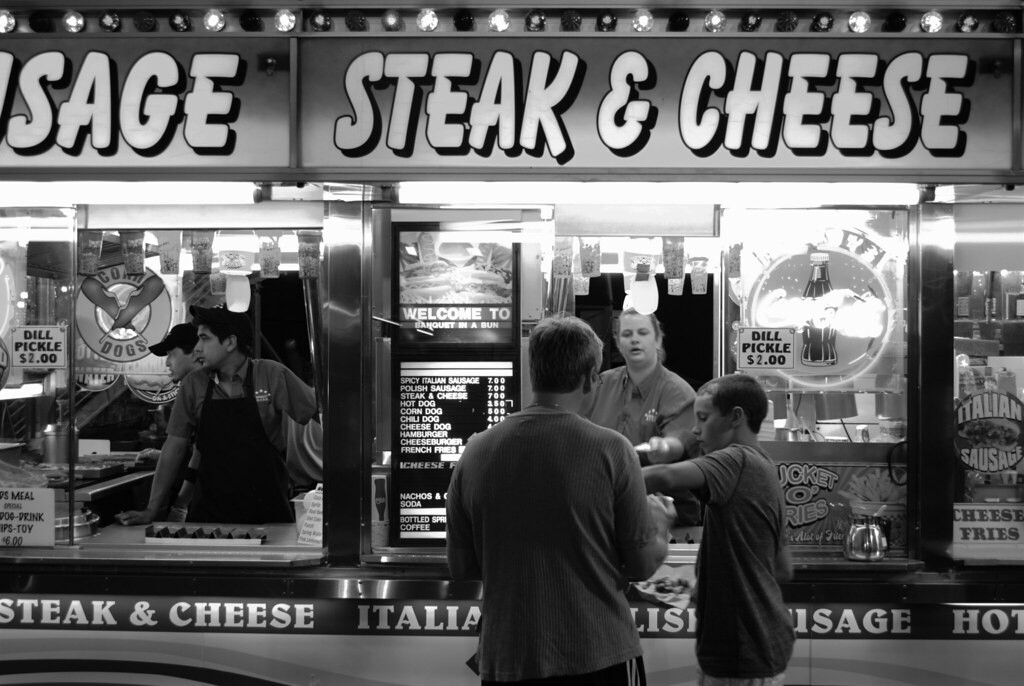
top-left (755, 399), bottom-right (777, 441)
top-left (372, 474), bottom-right (390, 548)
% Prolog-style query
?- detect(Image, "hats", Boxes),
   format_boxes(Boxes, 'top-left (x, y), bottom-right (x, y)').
top-left (189, 303), bottom-right (254, 341)
top-left (148, 324), bottom-right (196, 357)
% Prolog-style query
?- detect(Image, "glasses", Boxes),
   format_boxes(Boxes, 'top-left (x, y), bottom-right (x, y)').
top-left (594, 369), bottom-right (604, 389)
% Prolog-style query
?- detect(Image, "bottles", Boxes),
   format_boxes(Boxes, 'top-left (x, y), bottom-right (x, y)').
top-left (799, 252), bottom-right (838, 366)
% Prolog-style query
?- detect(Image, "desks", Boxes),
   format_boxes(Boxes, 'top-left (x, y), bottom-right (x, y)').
top-left (54, 452), bottom-right (156, 515)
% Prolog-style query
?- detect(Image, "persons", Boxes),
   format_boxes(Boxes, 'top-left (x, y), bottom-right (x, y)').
top-left (116, 306), bottom-right (321, 527)
top-left (584, 311), bottom-right (701, 525)
top-left (643, 374), bottom-right (794, 686)
top-left (446, 315), bottom-right (675, 685)
top-left (135, 323), bottom-right (203, 522)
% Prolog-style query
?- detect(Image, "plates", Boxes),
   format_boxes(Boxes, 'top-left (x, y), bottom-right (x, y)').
top-left (633, 587), bottom-right (695, 604)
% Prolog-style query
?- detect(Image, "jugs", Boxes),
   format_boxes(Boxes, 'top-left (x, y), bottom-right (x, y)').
top-left (842, 512), bottom-right (892, 562)
